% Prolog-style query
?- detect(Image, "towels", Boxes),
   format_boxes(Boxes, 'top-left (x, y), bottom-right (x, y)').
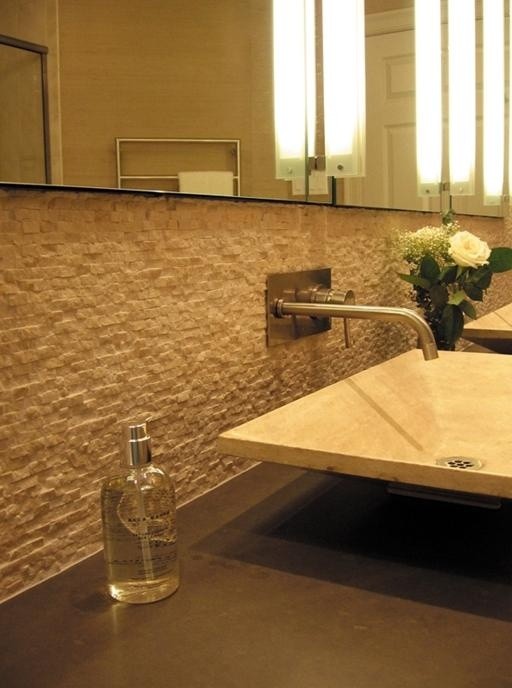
top-left (178, 170), bottom-right (234, 201)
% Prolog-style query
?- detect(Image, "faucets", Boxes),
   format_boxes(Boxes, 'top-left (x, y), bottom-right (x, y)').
top-left (268, 294), bottom-right (440, 363)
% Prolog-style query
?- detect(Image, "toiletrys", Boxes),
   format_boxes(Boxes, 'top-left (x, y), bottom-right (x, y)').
top-left (99, 412), bottom-right (180, 604)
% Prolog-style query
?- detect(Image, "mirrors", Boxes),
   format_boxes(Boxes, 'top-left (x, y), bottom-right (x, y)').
top-left (1, 0), bottom-right (509, 221)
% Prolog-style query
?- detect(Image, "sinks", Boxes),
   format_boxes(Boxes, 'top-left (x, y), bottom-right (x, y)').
top-left (217, 346), bottom-right (511, 498)
top-left (460, 302), bottom-right (512, 340)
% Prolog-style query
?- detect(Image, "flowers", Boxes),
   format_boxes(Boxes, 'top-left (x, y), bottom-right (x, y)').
top-left (387, 209), bottom-right (512, 347)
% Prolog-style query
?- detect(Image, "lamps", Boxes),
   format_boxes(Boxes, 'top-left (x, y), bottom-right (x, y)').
top-left (271, 1), bottom-right (317, 181)
top-left (448, 0), bottom-right (477, 197)
top-left (321, 1), bottom-right (367, 177)
top-left (414, 1), bottom-right (443, 197)
top-left (482, 1), bottom-right (506, 207)
